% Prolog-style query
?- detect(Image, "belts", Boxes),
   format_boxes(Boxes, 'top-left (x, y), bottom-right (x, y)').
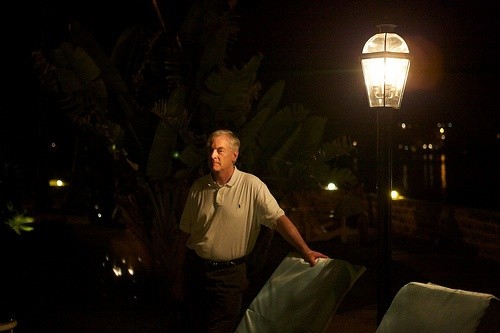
top-left (185, 249), bottom-right (246, 271)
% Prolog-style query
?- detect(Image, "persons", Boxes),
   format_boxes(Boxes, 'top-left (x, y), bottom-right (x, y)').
top-left (171, 129), bottom-right (330, 333)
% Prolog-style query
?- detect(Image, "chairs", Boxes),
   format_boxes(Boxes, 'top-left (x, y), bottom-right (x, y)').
top-left (235, 251), bottom-right (365, 333)
top-left (376, 283), bottom-right (500, 333)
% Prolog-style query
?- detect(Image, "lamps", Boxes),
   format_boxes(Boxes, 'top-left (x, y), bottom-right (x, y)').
top-left (360, 23), bottom-right (412, 328)
